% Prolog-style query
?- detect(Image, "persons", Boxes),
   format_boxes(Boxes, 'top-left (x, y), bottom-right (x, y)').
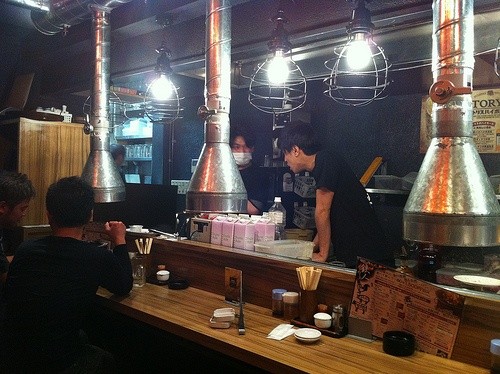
top-left (276, 121), bottom-right (397, 267)
top-left (228, 127), bottom-right (273, 216)
top-left (111, 146), bottom-right (127, 182)
top-left (0, 172), bottom-right (133, 374)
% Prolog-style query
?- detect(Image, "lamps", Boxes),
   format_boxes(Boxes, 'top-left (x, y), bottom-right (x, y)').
top-left (138, 16), bottom-right (185, 123)
top-left (81, 76), bottom-right (124, 130)
top-left (320, 1), bottom-right (394, 108)
top-left (247, 0), bottom-right (307, 115)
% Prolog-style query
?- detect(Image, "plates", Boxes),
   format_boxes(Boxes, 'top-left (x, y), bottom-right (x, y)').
top-left (452, 274), bottom-right (500, 287)
top-left (294, 327), bottom-right (322, 344)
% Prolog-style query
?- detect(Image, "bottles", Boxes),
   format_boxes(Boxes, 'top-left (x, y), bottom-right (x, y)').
top-left (272, 288), bottom-right (287, 317)
top-left (332, 305), bottom-right (345, 331)
top-left (282, 292), bottom-right (299, 321)
top-left (268, 196), bottom-right (287, 241)
top-left (122, 143), bottom-right (152, 158)
top-left (489, 339), bottom-right (500, 374)
top-left (263, 155), bottom-right (278, 167)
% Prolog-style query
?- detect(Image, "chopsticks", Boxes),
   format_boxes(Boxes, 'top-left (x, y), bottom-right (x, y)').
top-left (135, 238), bottom-right (153, 254)
top-left (296, 266), bottom-right (322, 290)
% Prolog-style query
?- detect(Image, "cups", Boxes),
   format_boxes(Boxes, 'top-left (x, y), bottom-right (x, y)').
top-left (132, 265), bottom-right (146, 288)
top-left (299, 288), bottom-right (320, 324)
top-left (313, 312), bottom-right (332, 328)
top-left (129, 224), bottom-right (143, 232)
top-left (156, 270), bottom-right (170, 281)
top-left (134, 254), bottom-right (152, 277)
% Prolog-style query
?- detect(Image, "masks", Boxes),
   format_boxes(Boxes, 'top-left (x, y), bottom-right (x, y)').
top-left (232, 152), bottom-right (252, 166)
